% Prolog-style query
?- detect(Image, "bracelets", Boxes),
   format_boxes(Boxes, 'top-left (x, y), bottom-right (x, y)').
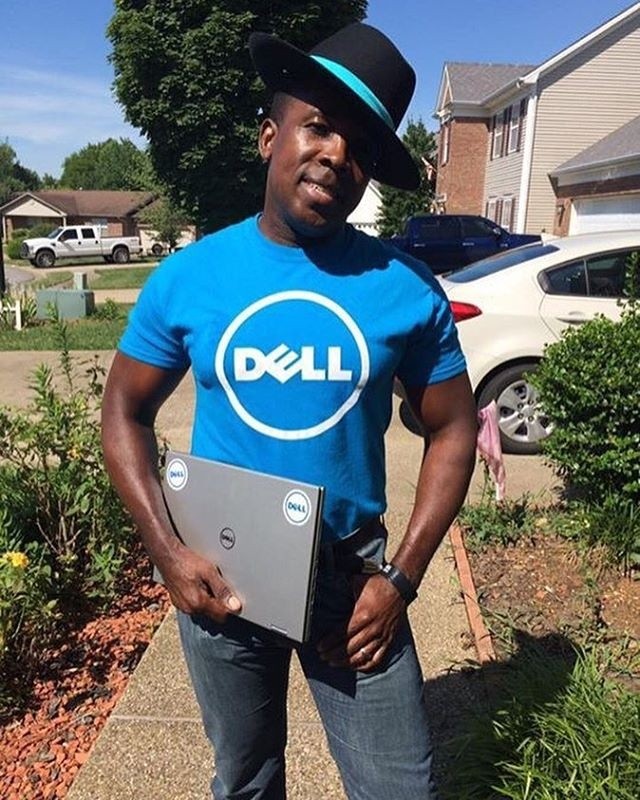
top-left (381, 564), bottom-right (418, 606)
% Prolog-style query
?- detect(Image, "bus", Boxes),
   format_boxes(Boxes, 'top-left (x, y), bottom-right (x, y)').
top-left (136, 224), bottom-right (198, 256)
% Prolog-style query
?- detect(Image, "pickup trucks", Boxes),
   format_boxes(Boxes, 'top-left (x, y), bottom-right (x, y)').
top-left (20, 225), bottom-right (140, 268)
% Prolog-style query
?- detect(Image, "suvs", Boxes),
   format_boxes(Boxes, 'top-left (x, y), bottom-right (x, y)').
top-left (381, 213), bottom-right (541, 276)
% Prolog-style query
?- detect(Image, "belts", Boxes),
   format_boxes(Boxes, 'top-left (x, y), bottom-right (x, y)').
top-left (318, 517), bottom-right (382, 561)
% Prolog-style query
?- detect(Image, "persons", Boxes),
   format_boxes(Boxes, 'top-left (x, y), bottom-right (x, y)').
top-left (100, 24), bottom-right (482, 800)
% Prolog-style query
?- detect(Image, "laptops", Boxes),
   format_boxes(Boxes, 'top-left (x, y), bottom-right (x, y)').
top-left (154, 453), bottom-right (326, 644)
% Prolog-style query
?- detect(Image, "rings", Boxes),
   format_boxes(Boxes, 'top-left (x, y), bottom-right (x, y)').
top-left (361, 647), bottom-right (371, 660)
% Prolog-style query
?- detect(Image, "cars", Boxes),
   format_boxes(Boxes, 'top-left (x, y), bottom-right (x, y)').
top-left (394, 230), bottom-right (640, 455)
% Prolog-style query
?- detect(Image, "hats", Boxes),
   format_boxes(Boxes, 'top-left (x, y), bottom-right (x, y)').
top-left (250, 22), bottom-right (423, 192)
